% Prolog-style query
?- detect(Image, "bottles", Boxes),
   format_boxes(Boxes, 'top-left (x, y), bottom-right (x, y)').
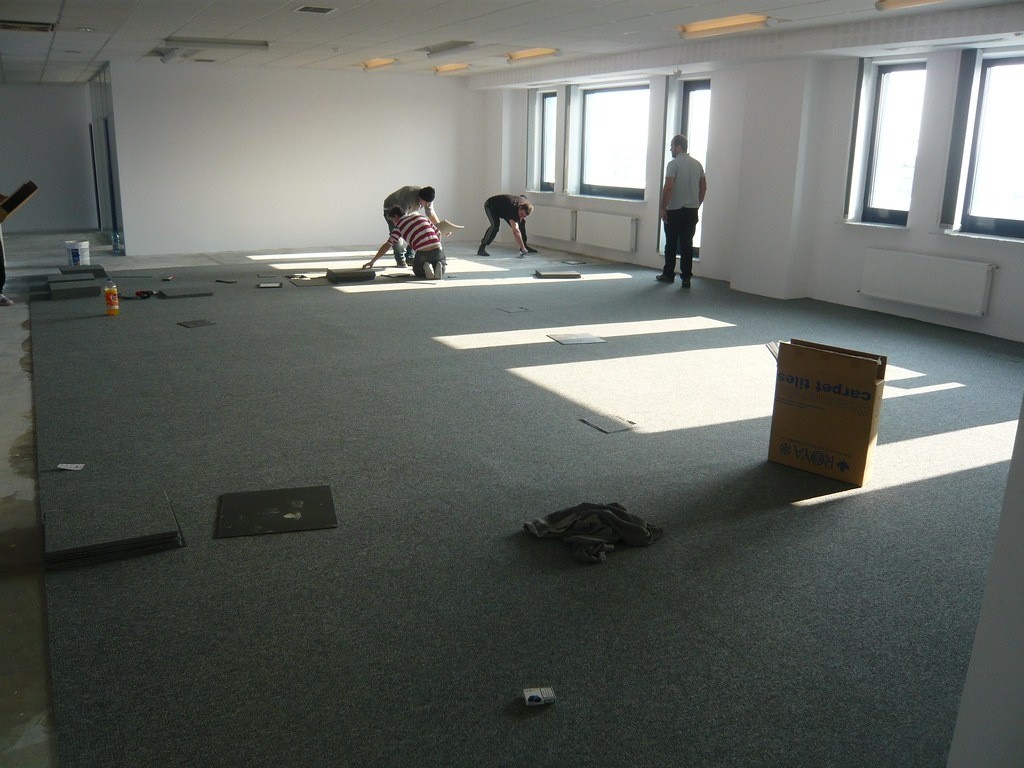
top-left (112, 231), bottom-right (120, 255)
top-left (104, 279), bottom-right (118, 315)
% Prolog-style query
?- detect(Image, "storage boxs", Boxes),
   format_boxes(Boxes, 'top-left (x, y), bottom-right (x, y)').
top-left (766, 337), bottom-right (888, 488)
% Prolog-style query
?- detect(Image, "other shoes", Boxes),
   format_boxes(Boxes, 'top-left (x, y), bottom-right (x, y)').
top-left (520, 245), bottom-right (537, 252)
top-left (682, 278), bottom-right (691, 287)
top-left (407, 258), bottom-right (414, 266)
top-left (397, 260), bottom-right (407, 267)
top-left (477, 249), bottom-right (489, 256)
top-left (656, 273), bottom-right (675, 283)
top-left (0, 294), bottom-right (14, 306)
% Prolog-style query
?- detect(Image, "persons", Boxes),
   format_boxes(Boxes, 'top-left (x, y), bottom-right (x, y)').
top-left (362, 185), bottom-right (453, 280)
top-left (656, 134), bottom-right (706, 289)
top-left (476, 194), bottom-right (538, 256)
top-left (0, 193), bottom-right (14, 306)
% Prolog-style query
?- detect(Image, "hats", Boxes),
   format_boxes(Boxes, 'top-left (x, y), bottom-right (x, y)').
top-left (419, 186), bottom-right (435, 202)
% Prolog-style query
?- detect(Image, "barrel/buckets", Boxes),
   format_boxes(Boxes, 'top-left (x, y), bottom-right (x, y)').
top-left (65, 240), bottom-right (90, 266)
